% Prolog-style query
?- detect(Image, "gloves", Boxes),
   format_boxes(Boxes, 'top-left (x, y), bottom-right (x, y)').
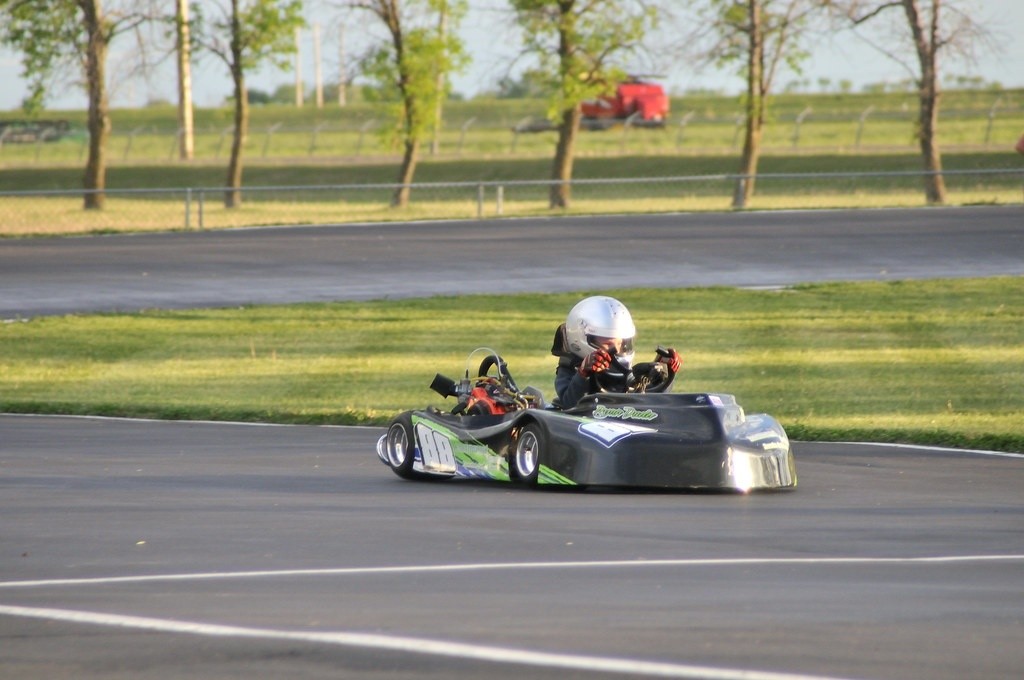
top-left (578, 348), bottom-right (612, 377)
top-left (655, 348), bottom-right (681, 374)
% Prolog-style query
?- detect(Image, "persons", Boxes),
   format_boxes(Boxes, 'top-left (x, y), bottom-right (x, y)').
top-left (555, 296), bottom-right (683, 410)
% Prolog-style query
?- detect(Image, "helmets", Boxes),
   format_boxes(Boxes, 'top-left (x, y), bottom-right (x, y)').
top-left (566, 295), bottom-right (635, 370)
